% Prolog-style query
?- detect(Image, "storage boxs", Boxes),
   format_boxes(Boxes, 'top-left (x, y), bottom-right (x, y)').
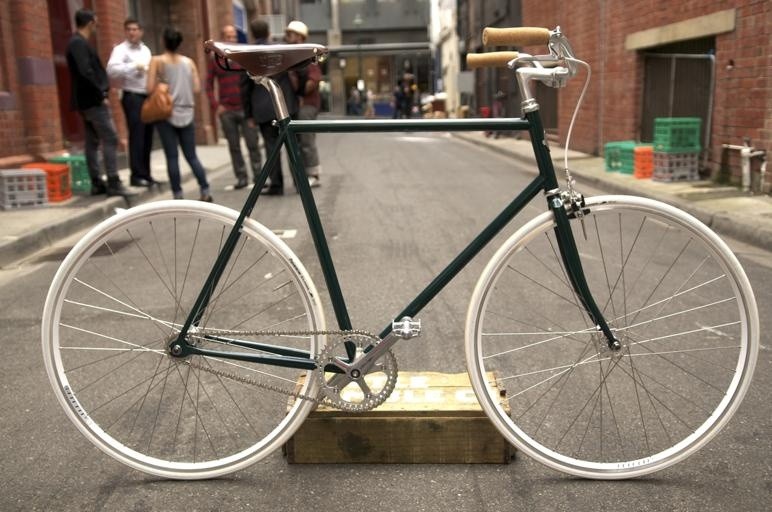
top-left (281, 371), bottom-right (518, 465)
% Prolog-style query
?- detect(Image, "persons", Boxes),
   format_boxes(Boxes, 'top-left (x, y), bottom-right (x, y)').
top-left (239, 20), bottom-right (309, 196)
top-left (64, 7), bottom-right (138, 197)
top-left (392, 73), bottom-right (415, 119)
top-left (348, 85), bottom-right (362, 116)
top-left (205, 24), bottom-right (268, 190)
top-left (105, 16), bottom-right (161, 188)
top-left (144, 25), bottom-right (213, 202)
top-left (363, 85), bottom-right (377, 119)
top-left (284, 20), bottom-right (321, 188)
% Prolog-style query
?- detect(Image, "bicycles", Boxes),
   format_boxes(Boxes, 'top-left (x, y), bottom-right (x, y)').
top-left (30, 23), bottom-right (764, 478)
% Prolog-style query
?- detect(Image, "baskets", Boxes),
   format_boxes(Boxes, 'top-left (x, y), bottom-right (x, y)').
top-left (604, 140), bottom-right (653, 175)
top-left (652, 115), bottom-right (702, 154)
top-left (20, 163), bottom-right (72, 203)
top-left (48, 153), bottom-right (93, 195)
top-left (0, 169), bottom-right (51, 212)
top-left (650, 150), bottom-right (701, 182)
top-left (633, 145), bottom-right (654, 178)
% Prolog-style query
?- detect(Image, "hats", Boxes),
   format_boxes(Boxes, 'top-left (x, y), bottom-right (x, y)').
top-left (285, 20), bottom-right (309, 39)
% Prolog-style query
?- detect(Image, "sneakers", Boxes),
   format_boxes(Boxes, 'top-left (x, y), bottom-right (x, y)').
top-left (198, 176), bottom-right (321, 202)
top-left (131, 176), bottom-right (158, 187)
top-left (106, 178), bottom-right (139, 197)
top-left (90, 180), bottom-right (108, 196)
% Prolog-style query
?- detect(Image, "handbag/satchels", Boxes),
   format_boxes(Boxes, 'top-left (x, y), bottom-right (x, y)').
top-left (139, 82), bottom-right (173, 124)
